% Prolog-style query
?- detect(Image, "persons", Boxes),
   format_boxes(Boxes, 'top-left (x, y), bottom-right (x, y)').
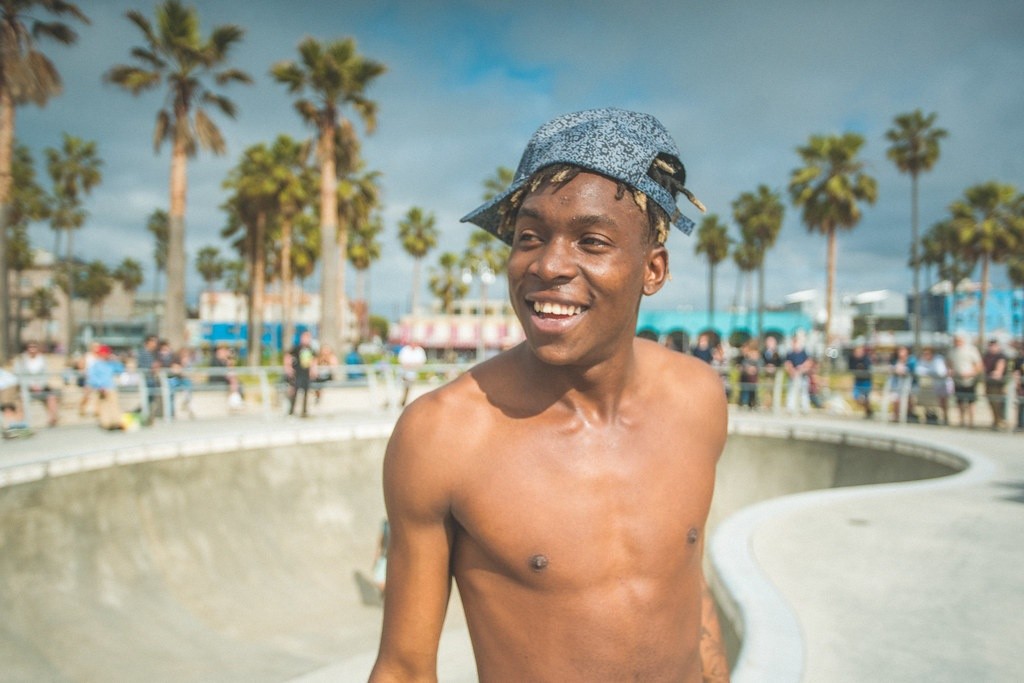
top-left (364, 103), bottom-right (737, 683)
top-left (2, 317), bottom-right (1024, 450)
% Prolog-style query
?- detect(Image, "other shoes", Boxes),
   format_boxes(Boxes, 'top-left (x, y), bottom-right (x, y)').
top-left (1013, 426), bottom-right (1024, 432)
top-left (994, 420), bottom-right (1008, 431)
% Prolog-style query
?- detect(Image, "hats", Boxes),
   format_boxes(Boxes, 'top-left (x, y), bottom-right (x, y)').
top-left (96, 346), bottom-right (110, 353)
top-left (459, 108), bottom-right (695, 248)
